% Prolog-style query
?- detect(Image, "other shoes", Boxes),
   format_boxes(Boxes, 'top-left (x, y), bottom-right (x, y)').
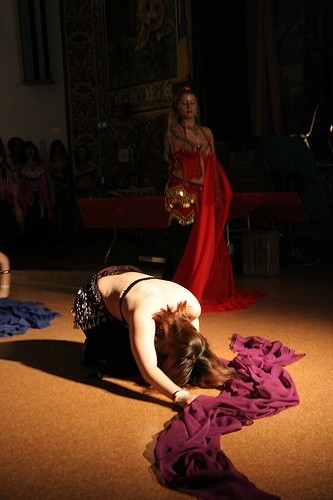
top-left (81, 358), bottom-right (105, 381)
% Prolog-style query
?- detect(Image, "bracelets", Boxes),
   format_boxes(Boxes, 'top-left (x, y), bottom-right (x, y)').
top-left (0, 270), bottom-right (11, 274)
top-left (171, 389), bottom-right (190, 407)
top-left (195, 142), bottom-right (208, 154)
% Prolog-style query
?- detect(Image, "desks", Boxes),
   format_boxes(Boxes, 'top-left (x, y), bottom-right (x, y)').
top-left (74, 192), bottom-right (299, 268)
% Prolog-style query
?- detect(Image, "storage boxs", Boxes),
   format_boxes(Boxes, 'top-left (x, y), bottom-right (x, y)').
top-left (242, 226), bottom-right (279, 279)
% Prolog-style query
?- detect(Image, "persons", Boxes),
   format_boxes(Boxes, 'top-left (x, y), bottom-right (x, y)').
top-left (164, 82), bottom-right (219, 295)
top-left (0, 129), bottom-right (169, 273)
top-left (0, 252), bottom-right (11, 298)
top-left (71, 265), bottom-right (236, 407)
